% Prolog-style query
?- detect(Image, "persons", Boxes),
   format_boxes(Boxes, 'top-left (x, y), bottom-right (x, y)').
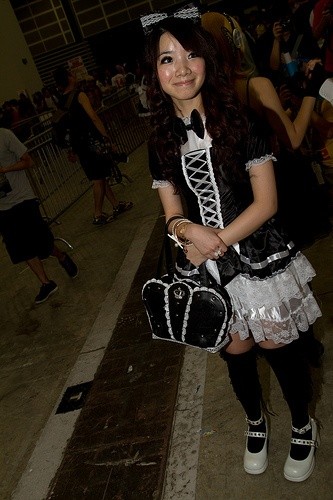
top-left (1, 0), bottom-right (333, 249)
top-left (0, 128), bottom-right (79, 303)
top-left (139, 8), bottom-right (323, 483)
top-left (55, 70), bottom-right (133, 225)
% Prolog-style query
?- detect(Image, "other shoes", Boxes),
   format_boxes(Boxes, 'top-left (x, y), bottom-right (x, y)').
top-left (58, 251), bottom-right (80, 279)
top-left (34, 279), bottom-right (58, 304)
top-left (92, 211), bottom-right (115, 227)
top-left (112, 200), bottom-right (133, 215)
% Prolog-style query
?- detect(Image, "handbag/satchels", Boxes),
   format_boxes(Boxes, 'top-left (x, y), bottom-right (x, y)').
top-left (139, 215), bottom-right (232, 353)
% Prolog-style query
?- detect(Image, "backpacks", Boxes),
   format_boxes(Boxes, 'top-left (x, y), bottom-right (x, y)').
top-left (51, 87), bottom-right (79, 164)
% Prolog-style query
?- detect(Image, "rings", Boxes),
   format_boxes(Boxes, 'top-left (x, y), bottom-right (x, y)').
top-left (214, 249), bottom-right (222, 256)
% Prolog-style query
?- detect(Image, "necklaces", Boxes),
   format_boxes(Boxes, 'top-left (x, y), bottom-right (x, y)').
top-left (174, 102), bottom-right (185, 117)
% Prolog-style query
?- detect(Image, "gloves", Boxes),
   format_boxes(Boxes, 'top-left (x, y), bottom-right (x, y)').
top-left (302, 63), bottom-right (332, 96)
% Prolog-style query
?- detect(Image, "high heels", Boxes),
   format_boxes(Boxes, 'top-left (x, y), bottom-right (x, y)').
top-left (283, 415), bottom-right (321, 482)
top-left (243, 409), bottom-right (270, 475)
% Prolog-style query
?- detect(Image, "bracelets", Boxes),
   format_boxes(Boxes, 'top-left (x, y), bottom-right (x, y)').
top-left (166, 214), bottom-right (195, 247)
